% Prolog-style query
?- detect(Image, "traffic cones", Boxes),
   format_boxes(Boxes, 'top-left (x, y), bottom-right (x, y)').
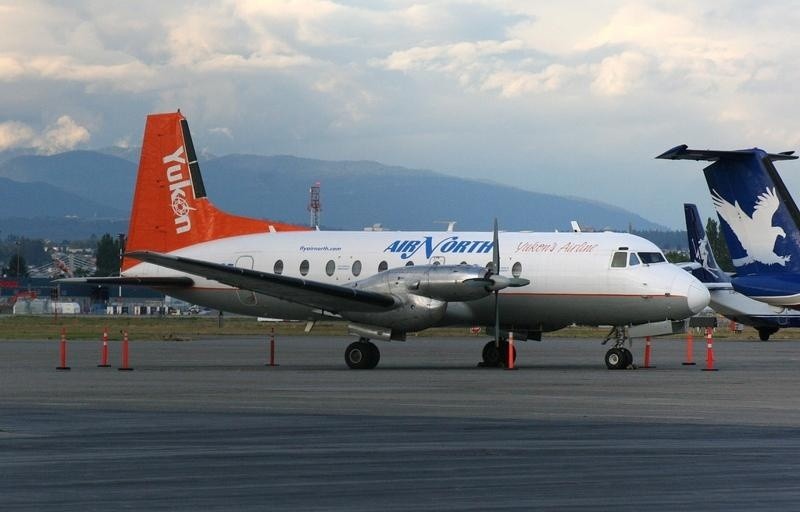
top-left (97, 328), bottom-right (112, 368)
top-left (682, 331), bottom-right (696, 365)
top-left (117, 331), bottom-right (134, 371)
top-left (55, 327), bottom-right (71, 371)
top-left (638, 337), bottom-right (656, 369)
top-left (264, 328), bottom-right (280, 367)
top-left (504, 333), bottom-right (519, 371)
top-left (701, 328), bottom-right (719, 372)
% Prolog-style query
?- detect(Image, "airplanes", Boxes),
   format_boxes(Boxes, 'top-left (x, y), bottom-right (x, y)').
top-left (655, 143), bottom-right (800, 342)
top-left (49, 109), bottom-right (732, 371)
